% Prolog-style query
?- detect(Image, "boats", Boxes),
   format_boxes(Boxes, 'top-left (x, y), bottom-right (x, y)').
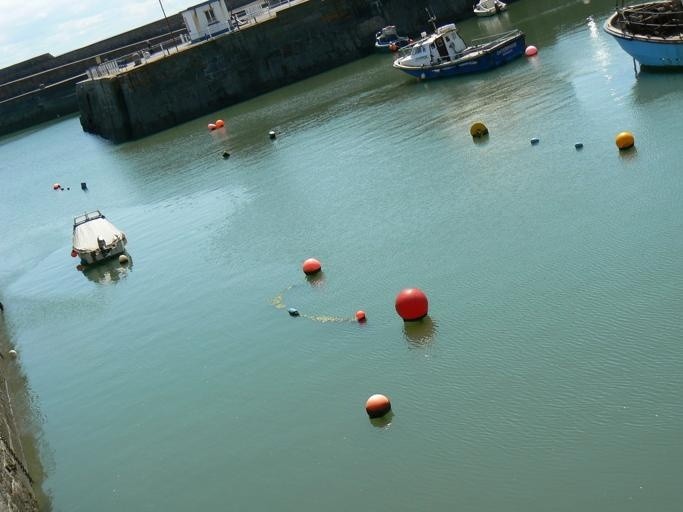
top-left (472, 0), bottom-right (506, 17)
top-left (605, 0), bottom-right (683, 65)
top-left (393, 23), bottom-right (527, 81)
top-left (374, 25), bottom-right (412, 51)
top-left (73, 210), bottom-right (126, 266)
